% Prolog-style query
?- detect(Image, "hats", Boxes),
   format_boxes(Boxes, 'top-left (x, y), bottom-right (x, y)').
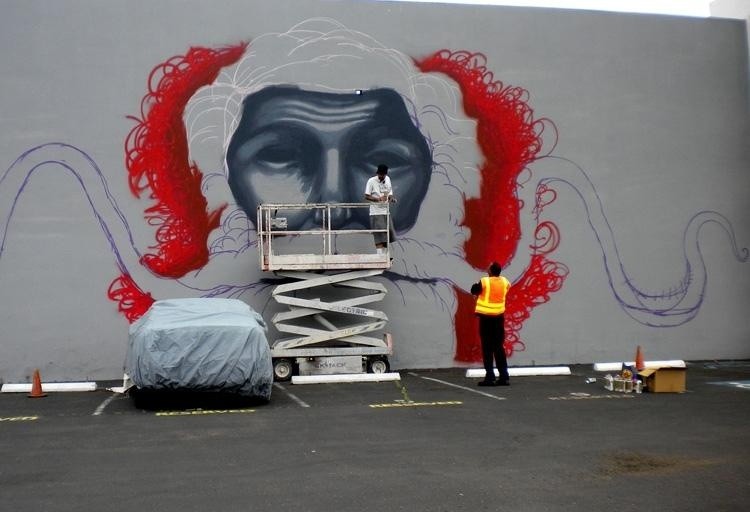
top-left (376, 163), bottom-right (388, 175)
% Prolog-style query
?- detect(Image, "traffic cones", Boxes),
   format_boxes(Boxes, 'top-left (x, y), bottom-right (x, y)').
top-left (26, 368), bottom-right (47, 398)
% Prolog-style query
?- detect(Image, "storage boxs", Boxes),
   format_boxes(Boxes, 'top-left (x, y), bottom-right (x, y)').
top-left (637, 364), bottom-right (688, 393)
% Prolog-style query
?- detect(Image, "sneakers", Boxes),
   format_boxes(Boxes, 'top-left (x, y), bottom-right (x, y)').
top-left (478, 378), bottom-right (511, 387)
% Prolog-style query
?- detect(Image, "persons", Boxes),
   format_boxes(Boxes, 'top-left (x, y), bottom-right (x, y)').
top-left (472, 262), bottom-right (512, 386)
top-left (227, 84), bottom-right (433, 244)
top-left (364, 163), bottom-right (397, 260)
